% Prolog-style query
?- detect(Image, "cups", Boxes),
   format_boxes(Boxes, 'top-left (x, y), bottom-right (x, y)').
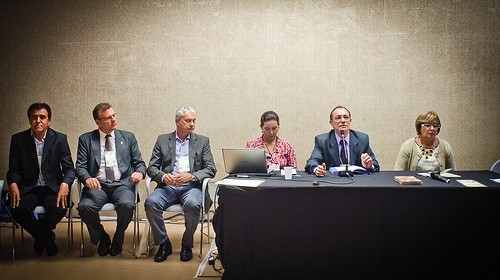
top-left (284, 167), bottom-right (293, 180)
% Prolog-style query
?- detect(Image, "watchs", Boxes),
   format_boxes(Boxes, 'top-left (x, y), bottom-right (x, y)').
top-left (191, 174), bottom-right (195, 182)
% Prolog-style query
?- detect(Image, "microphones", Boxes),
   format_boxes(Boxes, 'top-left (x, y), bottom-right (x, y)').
top-left (337, 130), bottom-right (354, 177)
top-left (430, 171), bottom-right (450, 182)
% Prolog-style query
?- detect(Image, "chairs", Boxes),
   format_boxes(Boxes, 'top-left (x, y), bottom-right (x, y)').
top-left (0, 176), bottom-right (75, 262)
top-left (77, 178), bottom-right (139, 257)
top-left (146, 176), bottom-right (210, 258)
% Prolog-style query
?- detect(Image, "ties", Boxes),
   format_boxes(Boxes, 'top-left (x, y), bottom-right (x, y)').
top-left (104, 133), bottom-right (114, 184)
top-left (340, 138), bottom-right (349, 164)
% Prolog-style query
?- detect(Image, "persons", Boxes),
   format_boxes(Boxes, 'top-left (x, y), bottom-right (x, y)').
top-left (243, 110), bottom-right (297, 170)
top-left (304, 106), bottom-right (381, 177)
top-left (394, 112), bottom-right (455, 170)
top-left (6, 103), bottom-right (75, 256)
top-left (73, 103), bottom-right (147, 256)
top-left (145, 106), bottom-right (217, 262)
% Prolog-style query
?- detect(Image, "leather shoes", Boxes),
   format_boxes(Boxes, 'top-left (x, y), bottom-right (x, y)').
top-left (180, 244), bottom-right (192, 261)
top-left (33, 237), bottom-right (45, 255)
top-left (97, 235), bottom-right (111, 257)
top-left (154, 244), bottom-right (172, 261)
top-left (109, 231), bottom-right (125, 256)
top-left (45, 232), bottom-right (59, 255)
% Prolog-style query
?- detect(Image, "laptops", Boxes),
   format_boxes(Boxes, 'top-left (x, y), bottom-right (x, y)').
top-left (222, 147), bottom-right (272, 174)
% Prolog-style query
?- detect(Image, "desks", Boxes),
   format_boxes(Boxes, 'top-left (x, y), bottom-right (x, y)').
top-left (211, 170), bottom-right (500, 280)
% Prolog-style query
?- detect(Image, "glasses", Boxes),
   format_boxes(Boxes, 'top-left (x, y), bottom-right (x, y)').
top-left (421, 123), bottom-right (441, 128)
top-left (99, 112), bottom-right (118, 122)
